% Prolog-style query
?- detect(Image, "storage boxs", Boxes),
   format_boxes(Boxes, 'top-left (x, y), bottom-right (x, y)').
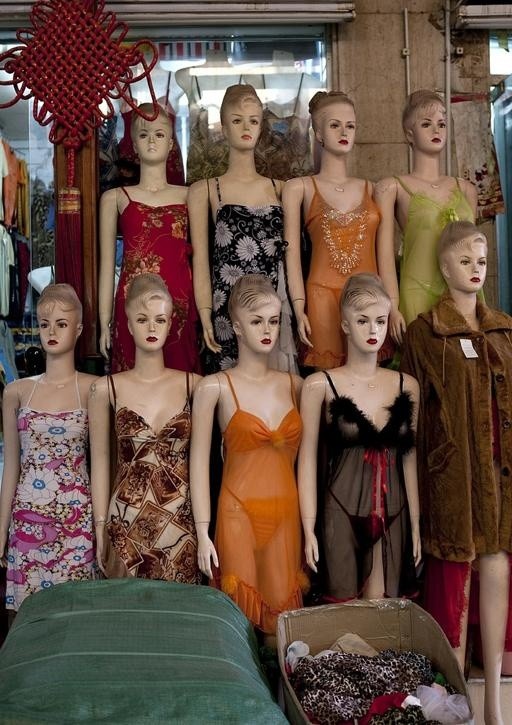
top-left (275, 598), bottom-right (474, 725)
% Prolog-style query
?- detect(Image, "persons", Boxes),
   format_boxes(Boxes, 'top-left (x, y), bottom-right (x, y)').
top-left (110, 47), bottom-right (187, 187)
top-left (95, 100), bottom-right (206, 378)
top-left (0, 280), bottom-right (102, 635)
top-left (397, 220), bottom-right (512, 725)
top-left (186, 270), bottom-right (316, 707)
top-left (372, 89), bottom-right (488, 371)
top-left (243, 48), bottom-right (326, 182)
top-left (174, 49), bottom-right (250, 186)
top-left (187, 83), bottom-right (303, 378)
top-left (85, 270), bottom-right (205, 590)
top-left (296, 272), bottom-right (425, 601)
top-left (280, 89), bottom-right (397, 374)
